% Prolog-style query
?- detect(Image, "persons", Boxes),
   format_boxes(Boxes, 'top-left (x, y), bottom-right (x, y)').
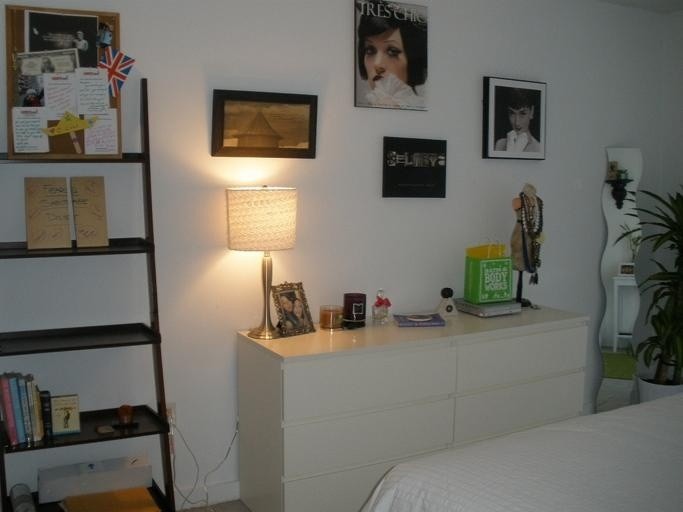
top-left (73, 31), bottom-right (89, 51)
top-left (495, 88), bottom-right (539, 153)
top-left (280, 296), bottom-right (305, 330)
top-left (23, 87), bottom-right (44, 107)
top-left (40, 57), bottom-right (54, 73)
top-left (510, 183), bottom-right (544, 272)
top-left (359, 4), bottom-right (427, 109)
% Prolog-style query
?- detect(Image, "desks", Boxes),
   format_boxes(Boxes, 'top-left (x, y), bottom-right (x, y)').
top-left (609, 275), bottom-right (639, 353)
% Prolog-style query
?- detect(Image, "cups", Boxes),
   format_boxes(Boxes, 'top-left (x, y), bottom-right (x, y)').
top-left (118, 405), bottom-right (133, 425)
top-left (318, 305), bottom-right (344, 332)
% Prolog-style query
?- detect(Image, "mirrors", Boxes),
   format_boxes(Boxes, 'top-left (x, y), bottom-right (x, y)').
top-left (595, 143), bottom-right (647, 355)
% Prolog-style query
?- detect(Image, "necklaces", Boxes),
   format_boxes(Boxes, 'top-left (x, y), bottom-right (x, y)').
top-left (520, 191), bottom-right (544, 285)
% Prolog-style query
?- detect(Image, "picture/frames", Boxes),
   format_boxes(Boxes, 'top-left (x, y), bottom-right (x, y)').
top-left (353, 1), bottom-right (432, 112)
top-left (208, 88), bottom-right (319, 160)
top-left (380, 135), bottom-right (446, 197)
top-left (4, 3), bottom-right (124, 161)
top-left (480, 75), bottom-right (547, 162)
top-left (269, 280), bottom-right (317, 337)
top-left (617, 262), bottom-right (635, 276)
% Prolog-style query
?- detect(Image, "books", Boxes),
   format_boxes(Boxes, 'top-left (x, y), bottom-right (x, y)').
top-left (0, 371), bottom-right (82, 446)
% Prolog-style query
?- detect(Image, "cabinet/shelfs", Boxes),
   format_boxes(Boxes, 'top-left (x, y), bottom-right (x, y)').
top-left (234, 294), bottom-right (593, 512)
top-left (0, 75), bottom-right (175, 512)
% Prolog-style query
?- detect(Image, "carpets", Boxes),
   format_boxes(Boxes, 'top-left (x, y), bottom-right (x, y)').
top-left (600, 347), bottom-right (638, 380)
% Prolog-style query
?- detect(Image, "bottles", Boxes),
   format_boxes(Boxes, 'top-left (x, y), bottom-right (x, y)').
top-left (371, 289), bottom-right (390, 322)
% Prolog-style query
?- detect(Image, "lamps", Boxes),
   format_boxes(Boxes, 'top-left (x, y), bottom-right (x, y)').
top-left (224, 182), bottom-right (300, 340)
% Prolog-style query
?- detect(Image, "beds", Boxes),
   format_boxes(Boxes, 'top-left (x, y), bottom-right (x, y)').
top-left (359, 392), bottom-right (681, 512)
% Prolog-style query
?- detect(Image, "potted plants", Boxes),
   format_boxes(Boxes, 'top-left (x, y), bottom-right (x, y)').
top-left (630, 184), bottom-right (683, 406)
